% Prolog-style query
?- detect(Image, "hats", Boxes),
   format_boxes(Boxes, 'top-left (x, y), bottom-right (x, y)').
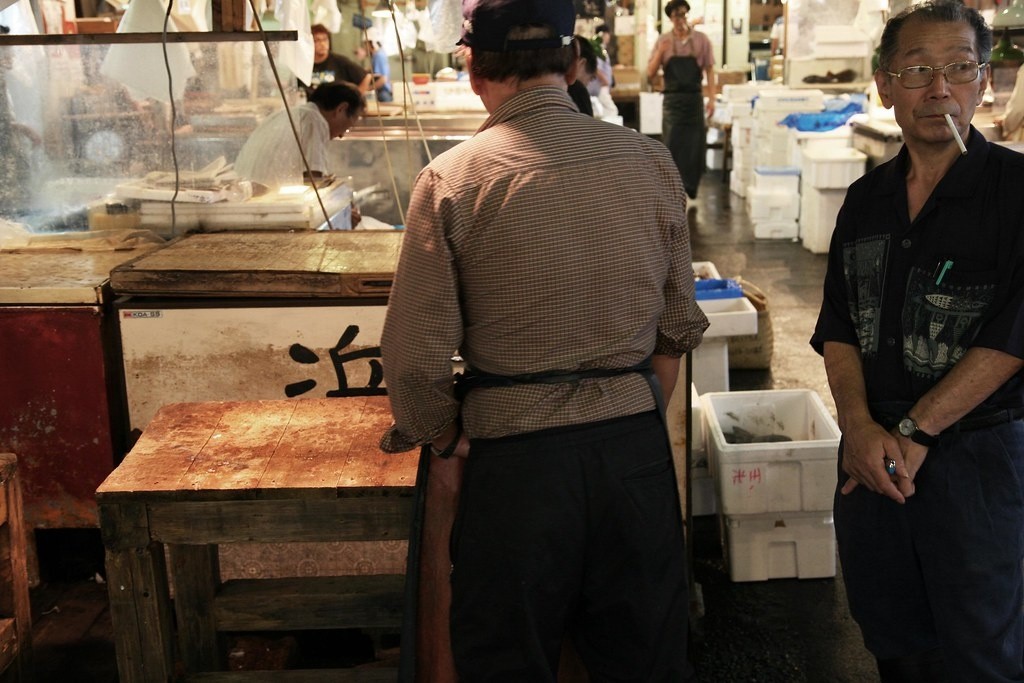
top-left (454, 0), bottom-right (576, 51)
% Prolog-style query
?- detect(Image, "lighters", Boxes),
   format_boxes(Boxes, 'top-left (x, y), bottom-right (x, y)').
top-left (886, 459), bottom-right (898, 473)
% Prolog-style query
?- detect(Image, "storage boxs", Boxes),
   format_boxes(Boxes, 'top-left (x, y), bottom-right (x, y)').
top-left (704, 85), bottom-right (904, 253)
top-left (691, 261), bottom-right (842, 581)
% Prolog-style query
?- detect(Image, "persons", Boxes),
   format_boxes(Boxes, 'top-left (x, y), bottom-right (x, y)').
top-left (807, 0), bottom-right (1024, 683)
top-left (233, 24), bottom-right (393, 235)
top-left (380, 0), bottom-right (710, 682)
top-left (569, 23), bottom-right (619, 119)
top-left (646, 1), bottom-right (715, 214)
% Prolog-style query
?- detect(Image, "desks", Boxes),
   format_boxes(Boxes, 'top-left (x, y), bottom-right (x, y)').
top-left (96, 395), bottom-right (686, 683)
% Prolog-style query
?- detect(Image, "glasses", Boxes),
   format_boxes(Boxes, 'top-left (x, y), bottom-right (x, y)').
top-left (884, 61), bottom-right (988, 89)
top-left (342, 110), bottom-right (355, 132)
top-left (313, 36), bottom-right (329, 44)
top-left (670, 12), bottom-right (688, 19)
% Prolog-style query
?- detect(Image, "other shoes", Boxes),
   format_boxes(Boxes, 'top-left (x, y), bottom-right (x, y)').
top-left (687, 198), bottom-right (698, 217)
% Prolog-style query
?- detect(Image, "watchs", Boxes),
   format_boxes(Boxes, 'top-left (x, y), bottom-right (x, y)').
top-left (898, 418), bottom-right (937, 449)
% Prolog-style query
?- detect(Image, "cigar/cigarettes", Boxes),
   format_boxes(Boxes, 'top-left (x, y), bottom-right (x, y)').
top-left (946, 114), bottom-right (968, 154)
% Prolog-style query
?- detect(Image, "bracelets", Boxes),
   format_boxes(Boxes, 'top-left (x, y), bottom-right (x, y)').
top-left (431, 431), bottom-right (462, 459)
